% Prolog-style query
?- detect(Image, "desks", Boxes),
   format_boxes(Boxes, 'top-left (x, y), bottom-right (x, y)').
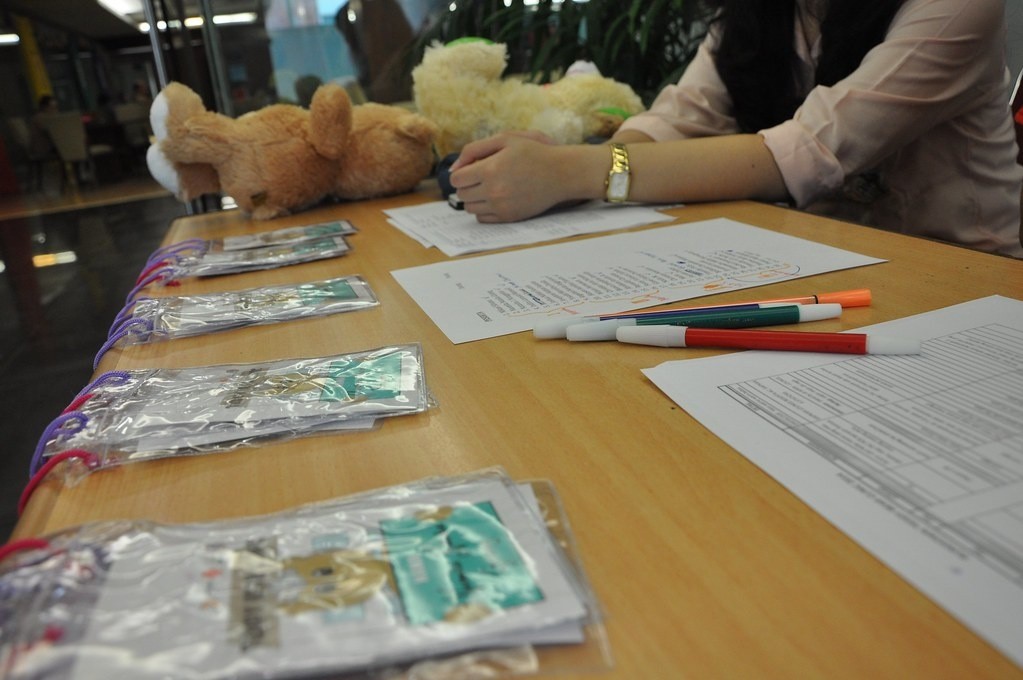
top-left (0, 180), bottom-right (1023, 680)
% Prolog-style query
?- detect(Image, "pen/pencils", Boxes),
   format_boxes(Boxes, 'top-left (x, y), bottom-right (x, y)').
top-left (565, 302), bottom-right (842, 341)
top-left (616, 324), bottom-right (922, 356)
top-left (582, 287), bottom-right (872, 317)
top-left (532, 302), bottom-right (802, 340)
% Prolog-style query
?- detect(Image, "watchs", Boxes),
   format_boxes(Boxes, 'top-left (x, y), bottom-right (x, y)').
top-left (604, 143), bottom-right (633, 203)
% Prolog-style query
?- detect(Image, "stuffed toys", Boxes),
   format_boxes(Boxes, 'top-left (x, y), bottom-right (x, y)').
top-left (146, 83), bottom-right (439, 221)
top-left (414, 37), bottom-right (645, 157)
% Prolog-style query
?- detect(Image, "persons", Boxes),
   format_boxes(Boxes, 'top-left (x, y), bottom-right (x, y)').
top-left (29, 95), bottom-right (68, 188)
top-left (448, 0), bottom-right (1023, 258)
top-left (89, 93), bottom-right (116, 125)
